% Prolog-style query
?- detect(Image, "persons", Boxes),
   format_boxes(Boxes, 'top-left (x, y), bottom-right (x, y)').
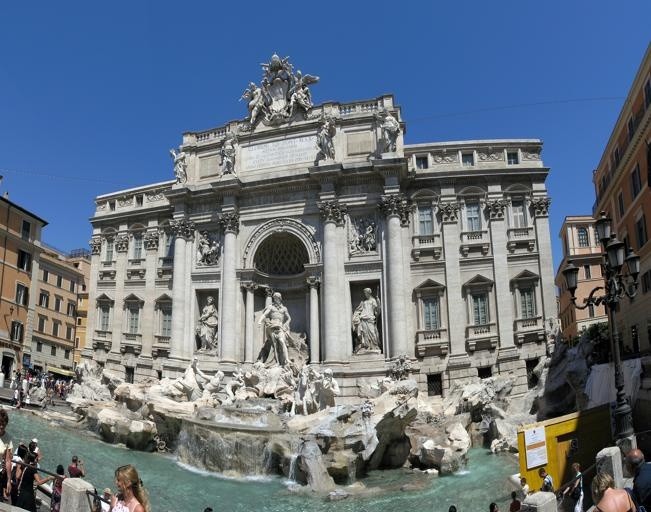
top-left (509, 491), bottom-right (520, 512)
top-left (538, 467), bottom-right (554, 491)
top-left (624, 448), bottom-right (651, 511)
top-left (196, 294), bottom-right (218, 351)
top-left (590, 472), bottom-right (637, 512)
top-left (449, 505), bottom-right (457, 512)
top-left (352, 287), bottom-right (382, 350)
top-left (258, 292), bottom-right (291, 364)
top-left (519, 478), bottom-right (529, 498)
top-left (0, 365), bottom-right (151, 512)
top-left (567, 461), bottom-right (586, 512)
top-left (171, 357), bottom-right (339, 416)
top-left (489, 503), bottom-right (500, 512)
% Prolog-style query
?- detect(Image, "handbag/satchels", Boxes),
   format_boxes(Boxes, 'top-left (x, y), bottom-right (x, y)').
top-left (570, 487), bottom-right (581, 500)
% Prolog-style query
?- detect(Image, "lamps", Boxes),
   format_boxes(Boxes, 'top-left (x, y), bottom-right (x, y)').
top-left (2, 305), bottom-right (14, 318)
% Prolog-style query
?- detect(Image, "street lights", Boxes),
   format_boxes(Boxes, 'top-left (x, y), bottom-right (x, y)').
top-left (562, 208), bottom-right (642, 436)
